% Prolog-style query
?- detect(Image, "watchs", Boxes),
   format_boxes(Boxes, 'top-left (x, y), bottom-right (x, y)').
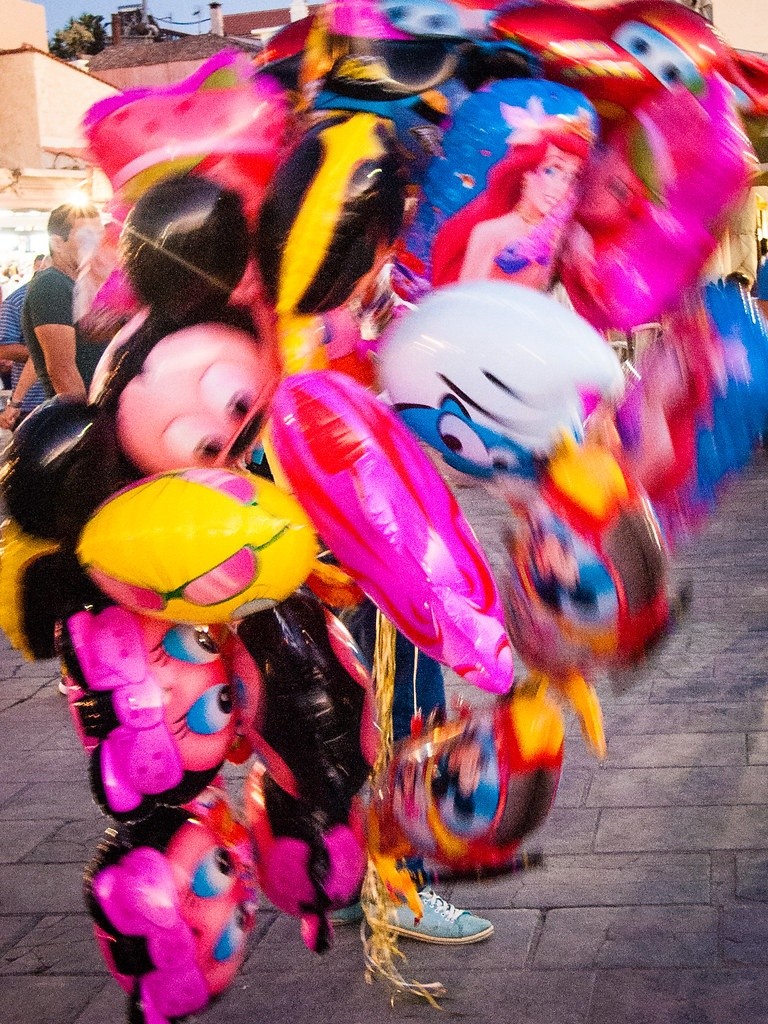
top-left (7, 398), bottom-right (23, 408)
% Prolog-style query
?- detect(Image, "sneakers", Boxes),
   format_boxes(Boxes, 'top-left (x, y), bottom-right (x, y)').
top-left (368, 885), bottom-right (494, 945)
top-left (326, 902), bottom-right (365, 927)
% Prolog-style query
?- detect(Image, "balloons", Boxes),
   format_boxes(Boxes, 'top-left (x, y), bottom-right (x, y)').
top-left (0, 0), bottom-right (768, 1024)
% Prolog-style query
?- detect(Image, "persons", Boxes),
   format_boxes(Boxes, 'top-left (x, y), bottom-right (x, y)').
top-left (298, 533), bottom-right (494, 946)
top-left (0, 203), bottom-right (113, 435)
